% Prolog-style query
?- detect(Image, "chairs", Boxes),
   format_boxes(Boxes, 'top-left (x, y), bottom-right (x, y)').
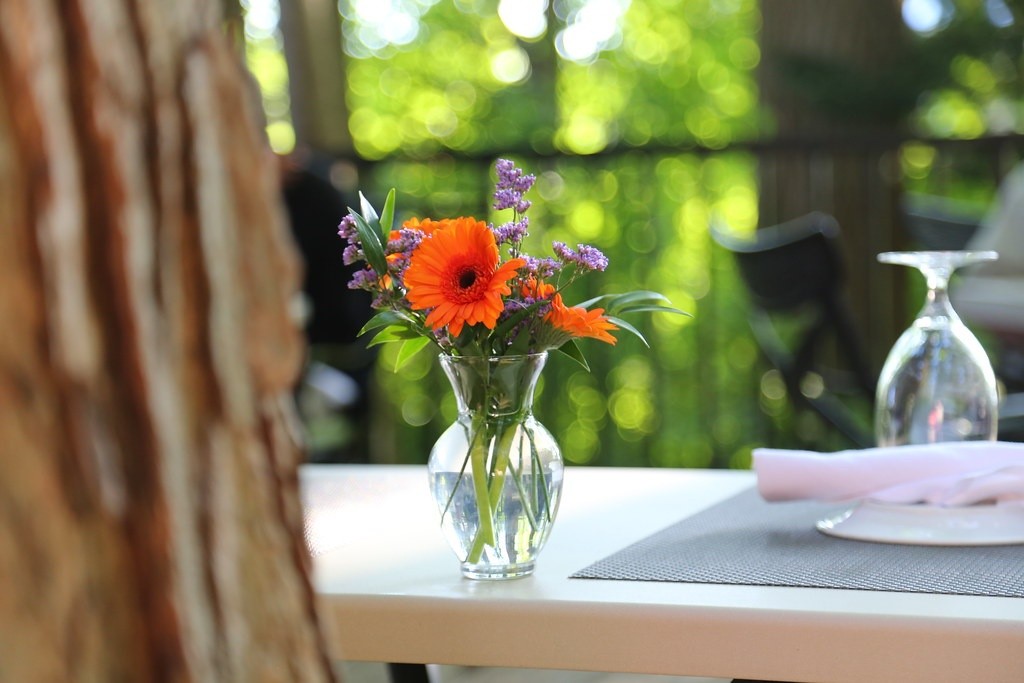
top-left (711, 213), bottom-right (1024, 448)
top-left (295, 171), bottom-right (432, 683)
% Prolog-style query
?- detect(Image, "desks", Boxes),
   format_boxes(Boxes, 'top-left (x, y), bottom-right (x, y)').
top-left (297, 465), bottom-right (1024, 683)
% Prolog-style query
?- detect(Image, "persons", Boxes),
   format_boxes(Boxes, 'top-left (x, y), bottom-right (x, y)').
top-left (279, 169), bottom-right (377, 464)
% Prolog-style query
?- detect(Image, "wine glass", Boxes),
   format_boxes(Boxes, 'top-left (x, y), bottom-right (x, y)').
top-left (874, 249), bottom-right (999, 448)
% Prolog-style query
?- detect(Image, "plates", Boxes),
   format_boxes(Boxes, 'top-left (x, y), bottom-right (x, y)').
top-left (815, 498), bottom-right (1024, 546)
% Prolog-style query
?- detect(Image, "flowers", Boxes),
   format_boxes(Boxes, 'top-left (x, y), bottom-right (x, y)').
top-left (339, 159), bottom-right (696, 566)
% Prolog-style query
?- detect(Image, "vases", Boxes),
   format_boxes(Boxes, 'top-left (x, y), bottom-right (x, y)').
top-left (427, 350), bottom-right (563, 581)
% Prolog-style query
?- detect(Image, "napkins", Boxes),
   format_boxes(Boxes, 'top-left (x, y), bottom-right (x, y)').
top-left (751, 442), bottom-right (1024, 506)
